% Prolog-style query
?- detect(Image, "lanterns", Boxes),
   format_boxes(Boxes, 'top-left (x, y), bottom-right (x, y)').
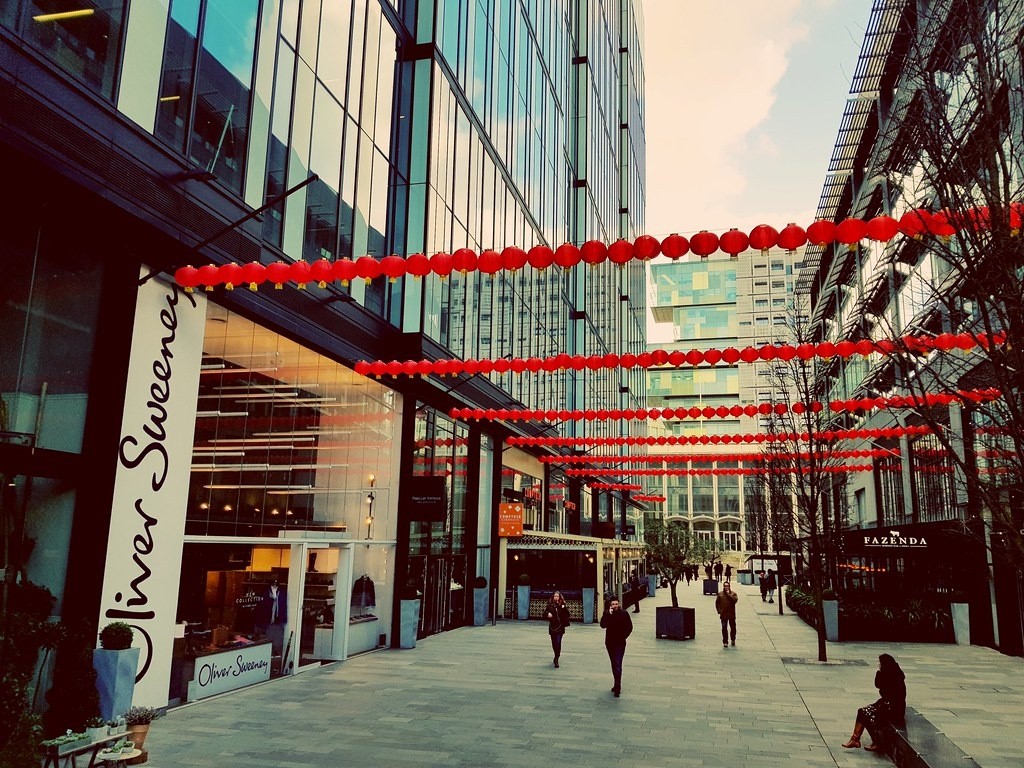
top-left (587, 482), bottom-right (667, 503)
top-left (353, 330), bottom-right (1009, 379)
top-left (447, 386), bottom-right (1024, 477)
top-left (173, 199), bottom-right (1024, 295)
top-left (501, 468), bottom-right (568, 500)
top-left (412, 437), bottom-right (468, 477)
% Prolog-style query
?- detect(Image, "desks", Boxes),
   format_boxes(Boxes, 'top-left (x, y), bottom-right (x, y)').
top-left (44, 729), bottom-right (133, 768)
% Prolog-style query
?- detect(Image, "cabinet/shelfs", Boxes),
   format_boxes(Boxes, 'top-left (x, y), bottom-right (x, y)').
top-left (301, 571), bottom-right (340, 653)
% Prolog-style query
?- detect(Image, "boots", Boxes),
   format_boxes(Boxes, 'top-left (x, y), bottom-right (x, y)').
top-left (864, 724), bottom-right (880, 751)
top-left (842, 721), bottom-right (864, 748)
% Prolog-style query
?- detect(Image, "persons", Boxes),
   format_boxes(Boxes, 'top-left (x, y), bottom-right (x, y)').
top-left (542, 591), bottom-right (571, 668)
top-left (714, 561), bottom-right (734, 582)
top-left (759, 569), bottom-right (777, 603)
top-left (716, 582), bottom-right (738, 647)
top-left (706, 564), bottom-right (711, 576)
top-left (258, 584), bottom-right (287, 643)
top-left (842, 653), bottom-right (907, 752)
top-left (351, 574), bottom-right (375, 607)
top-left (603, 566), bottom-right (607, 592)
top-left (600, 596), bottom-right (633, 697)
top-left (628, 570), bottom-right (640, 613)
top-left (679, 563), bottom-right (699, 586)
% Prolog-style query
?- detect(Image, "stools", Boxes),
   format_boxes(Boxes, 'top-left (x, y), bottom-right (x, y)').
top-left (95, 747), bottom-right (142, 768)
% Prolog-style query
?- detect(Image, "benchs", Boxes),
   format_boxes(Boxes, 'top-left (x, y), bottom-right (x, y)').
top-left (880, 708), bottom-right (983, 768)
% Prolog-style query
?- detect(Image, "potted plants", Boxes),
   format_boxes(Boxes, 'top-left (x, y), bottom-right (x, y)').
top-left (688, 534), bottom-right (731, 595)
top-left (636, 517), bottom-right (716, 641)
top-left (125, 705), bottom-right (165, 748)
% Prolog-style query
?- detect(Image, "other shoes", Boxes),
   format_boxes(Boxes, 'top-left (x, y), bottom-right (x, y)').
top-left (731, 641), bottom-right (735, 645)
top-left (724, 644), bottom-right (728, 647)
top-left (611, 686), bottom-right (621, 697)
top-left (554, 658), bottom-right (559, 668)
